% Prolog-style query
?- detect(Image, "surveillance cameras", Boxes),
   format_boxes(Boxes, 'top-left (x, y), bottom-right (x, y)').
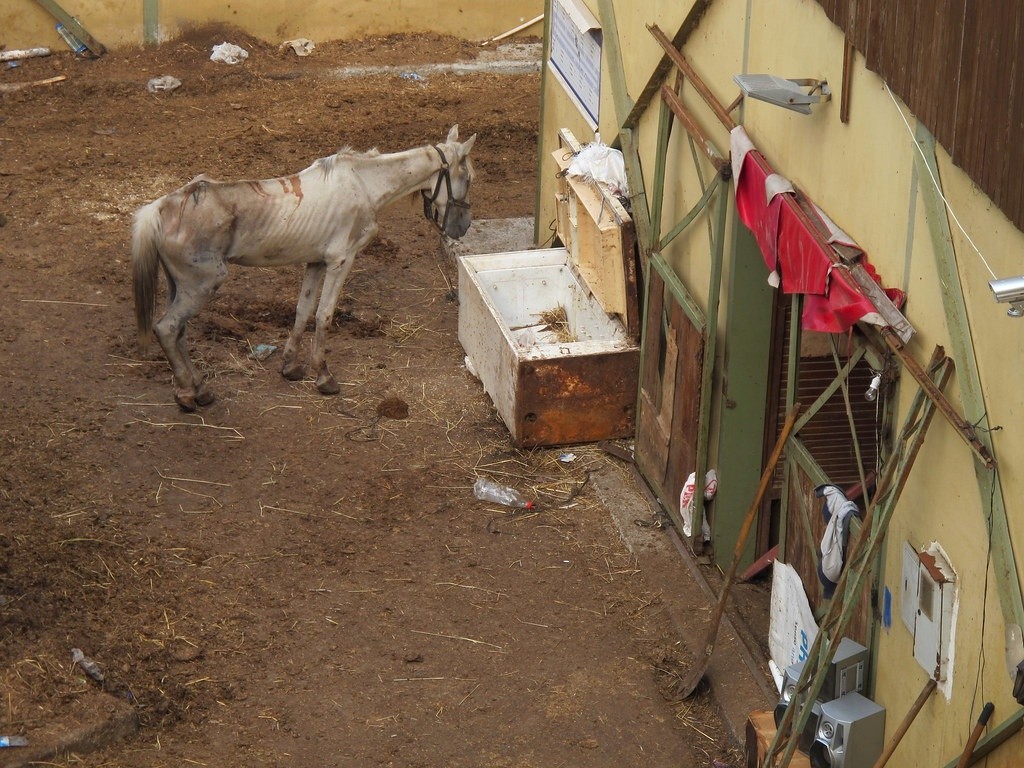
top-left (988, 277), bottom-right (1024, 318)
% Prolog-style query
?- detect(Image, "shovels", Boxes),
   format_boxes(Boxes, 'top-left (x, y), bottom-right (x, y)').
top-left (677, 403), bottom-right (798, 700)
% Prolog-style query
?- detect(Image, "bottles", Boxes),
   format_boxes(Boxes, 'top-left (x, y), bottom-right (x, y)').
top-left (474, 478), bottom-right (531, 509)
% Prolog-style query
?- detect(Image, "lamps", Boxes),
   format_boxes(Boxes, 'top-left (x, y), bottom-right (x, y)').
top-left (989, 277), bottom-right (1024, 317)
top-left (732, 73), bottom-right (814, 116)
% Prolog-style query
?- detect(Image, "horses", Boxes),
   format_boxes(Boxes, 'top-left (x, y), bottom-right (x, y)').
top-left (131, 123), bottom-right (478, 409)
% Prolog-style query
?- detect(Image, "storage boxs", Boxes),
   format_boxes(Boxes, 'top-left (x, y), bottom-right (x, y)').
top-left (457, 245), bottom-right (640, 450)
